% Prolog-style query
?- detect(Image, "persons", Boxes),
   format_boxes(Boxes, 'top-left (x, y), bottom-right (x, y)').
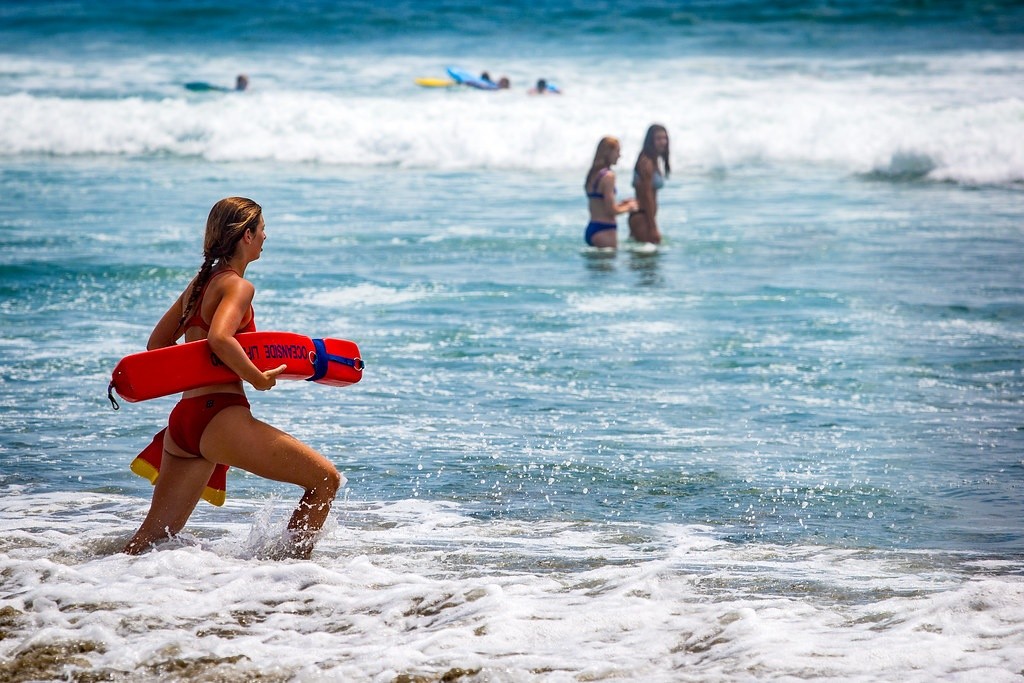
top-left (124, 196), bottom-right (340, 561)
top-left (527, 80), bottom-right (548, 95)
top-left (481, 74), bottom-right (508, 88)
top-left (628, 124), bottom-right (670, 244)
top-left (237, 77), bottom-right (247, 90)
top-left (584, 136), bottom-right (639, 249)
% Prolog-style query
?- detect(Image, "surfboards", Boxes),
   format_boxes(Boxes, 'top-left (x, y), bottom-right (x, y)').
top-left (545, 84), bottom-right (560, 93)
top-left (447, 67), bottom-right (505, 90)
top-left (185, 81), bottom-right (234, 92)
top-left (415, 78), bottom-right (454, 86)
